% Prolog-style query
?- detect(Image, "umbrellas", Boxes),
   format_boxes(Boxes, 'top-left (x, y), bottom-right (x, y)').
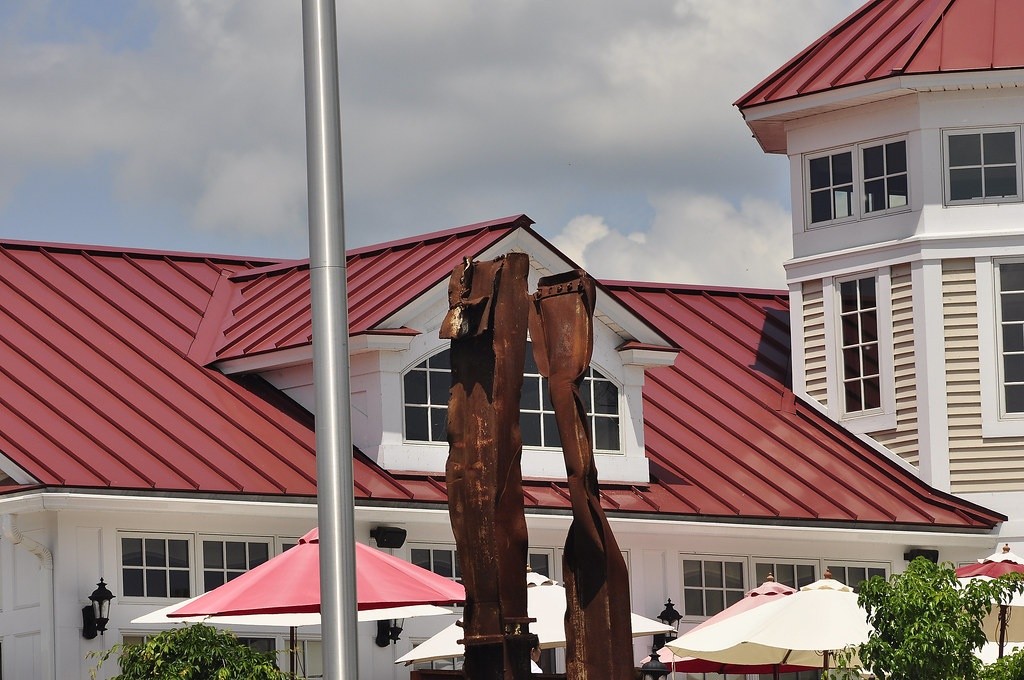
top-left (663, 568), bottom-right (882, 680)
top-left (948, 544), bottom-right (1024, 659)
top-left (640, 573), bottom-right (818, 680)
top-left (396, 563), bottom-right (676, 667)
top-left (130, 591), bottom-right (453, 680)
top-left (166, 527), bottom-right (466, 619)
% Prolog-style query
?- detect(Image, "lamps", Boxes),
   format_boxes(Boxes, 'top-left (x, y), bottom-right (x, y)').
top-left (375, 618), bottom-right (405, 648)
top-left (903, 549), bottom-right (939, 564)
top-left (653, 597), bottom-right (683, 649)
top-left (370, 525), bottom-right (407, 549)
top-left (82, 578), bottom-right (116, 640)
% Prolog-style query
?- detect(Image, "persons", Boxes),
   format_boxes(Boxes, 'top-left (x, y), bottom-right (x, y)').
top-left (529, 633), bottom-right (544, 673)
